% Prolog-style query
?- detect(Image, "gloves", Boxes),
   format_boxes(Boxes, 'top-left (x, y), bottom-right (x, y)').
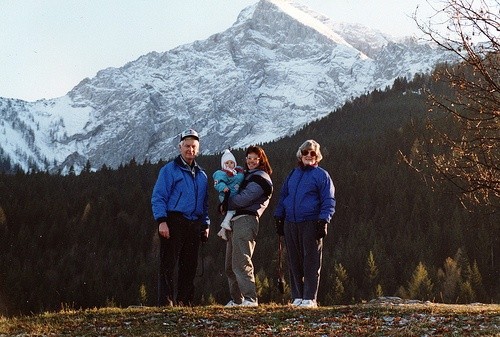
top-left (315, 222), bottom-right (328, 239)
top-left (273, 218), bottom-right (285, 236)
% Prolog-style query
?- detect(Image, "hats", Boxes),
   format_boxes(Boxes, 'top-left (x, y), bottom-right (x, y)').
top-left (179, 129), bottom-right (199, 142)
top-left (221, 149), bottom-right (237, 176)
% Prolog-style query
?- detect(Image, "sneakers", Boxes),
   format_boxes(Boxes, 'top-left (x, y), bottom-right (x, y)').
top-left (225, 301), bottom-right (242, 307)
top-left (239, 298), bottom-right (258, 307)
top-left (290, 299), bottom-right (302, 306)
top-left (300, 299), bottom-right (318, 307)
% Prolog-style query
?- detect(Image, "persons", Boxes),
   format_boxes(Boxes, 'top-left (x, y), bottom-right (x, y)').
top-left (274, 139), bottom-right (336, 308)
top-left (213, 149), bottom-right (245, 240)
top-left (224, 146), bottom-right (273, 307)
top-left (151, 129), bottom-right (210, 307)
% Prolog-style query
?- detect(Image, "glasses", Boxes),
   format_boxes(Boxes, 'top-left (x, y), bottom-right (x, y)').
top-left (246, 157), bottom-right (260, 161)
top-left (301, 150), bottom-right (316, 156)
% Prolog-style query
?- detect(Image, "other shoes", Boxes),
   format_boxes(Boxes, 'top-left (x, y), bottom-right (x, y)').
top-left (220, 222), bottom-right (232, 231)
top-left (217, 231), bottom-right (227, 240)
